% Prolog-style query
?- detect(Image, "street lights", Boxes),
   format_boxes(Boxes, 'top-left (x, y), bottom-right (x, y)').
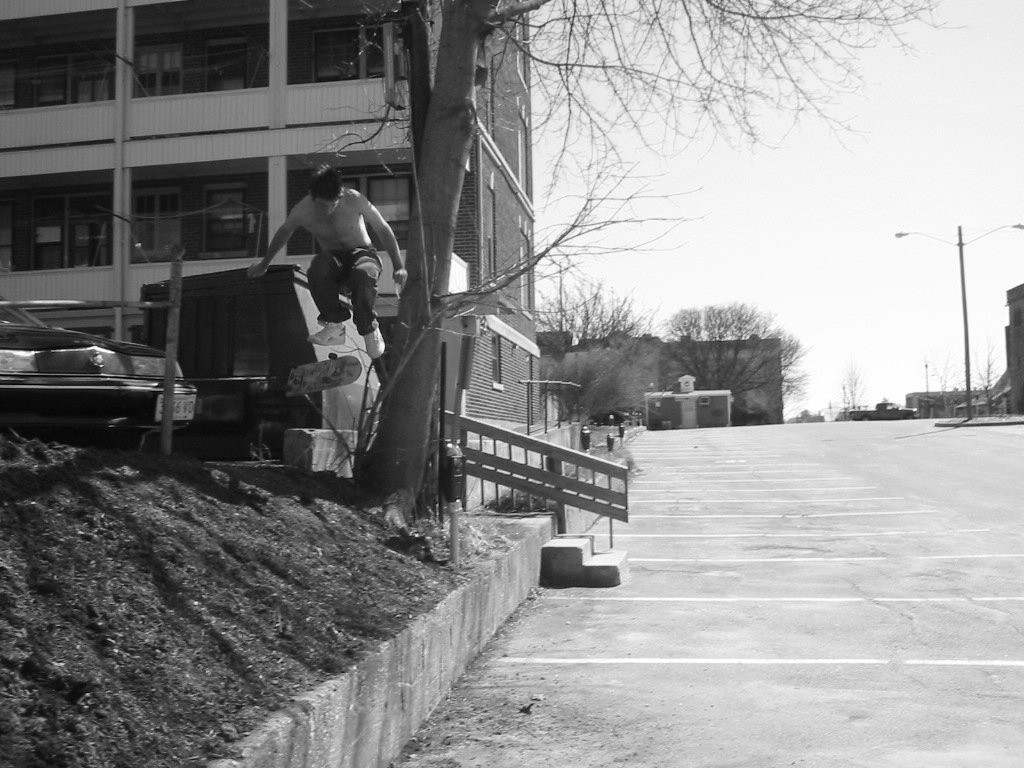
top-left (895, 223), bottom-right (1024, 418)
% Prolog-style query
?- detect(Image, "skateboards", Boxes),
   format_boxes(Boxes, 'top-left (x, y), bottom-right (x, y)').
top-left (285, 352), bottom-right (362, 397)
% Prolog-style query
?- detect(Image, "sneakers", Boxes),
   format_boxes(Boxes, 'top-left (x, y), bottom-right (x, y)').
top-left (307, 321), bottom-right (346, 345)
top-left (364, 327), bottom-right (385, 358)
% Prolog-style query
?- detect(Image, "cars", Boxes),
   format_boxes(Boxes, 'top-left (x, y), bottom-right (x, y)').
top-left (0, 290), bottom-right (197, 458)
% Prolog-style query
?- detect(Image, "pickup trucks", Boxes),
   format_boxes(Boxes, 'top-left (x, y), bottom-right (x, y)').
top-left (848, 402), bottom-right (921, 421)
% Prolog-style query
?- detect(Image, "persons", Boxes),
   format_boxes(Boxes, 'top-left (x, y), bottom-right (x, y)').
top-left (246, 165), bottom-right (408, 359)
